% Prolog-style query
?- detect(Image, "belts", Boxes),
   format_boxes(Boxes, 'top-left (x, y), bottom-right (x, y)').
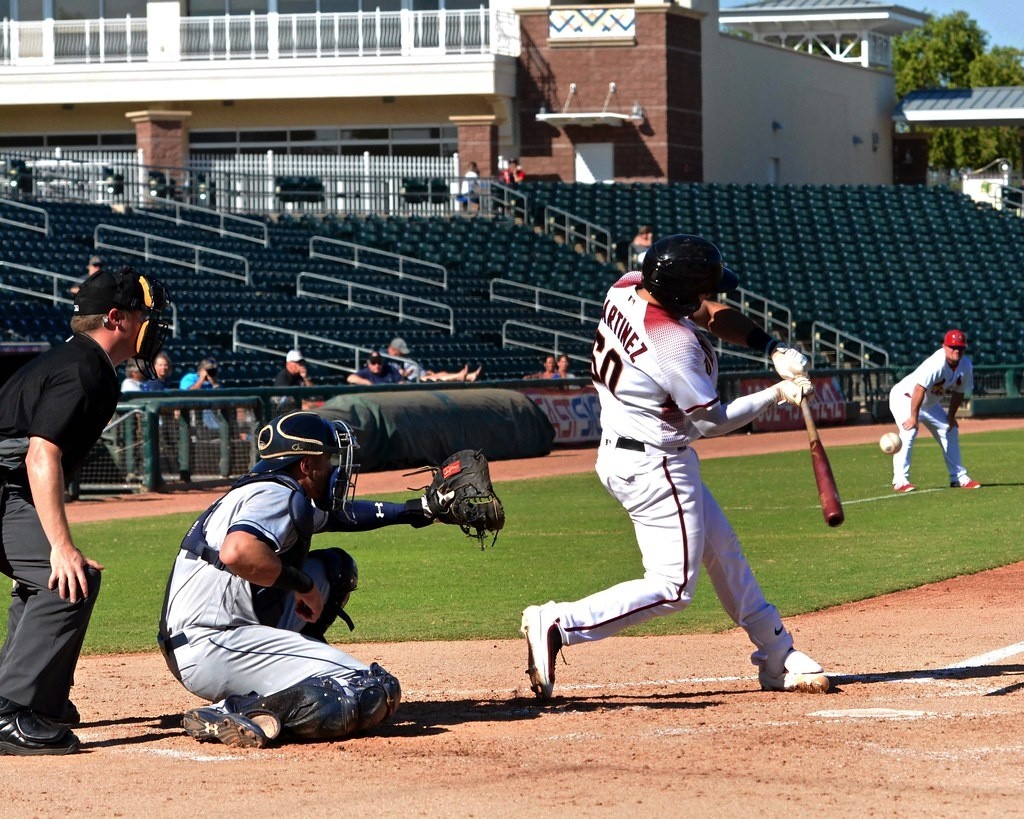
top-left (602, 435), bottom-right (688, 452)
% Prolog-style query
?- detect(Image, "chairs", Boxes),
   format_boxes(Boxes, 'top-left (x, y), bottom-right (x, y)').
top-left (0, 173), bottom-right (1024, 401)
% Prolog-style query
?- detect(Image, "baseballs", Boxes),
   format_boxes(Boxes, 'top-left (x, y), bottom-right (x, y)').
top-left (878, 432), bottom-right (903, 454)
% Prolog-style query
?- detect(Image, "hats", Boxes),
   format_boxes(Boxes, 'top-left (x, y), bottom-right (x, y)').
top-left (390, 338), bottom-right (411, 354)
top-left (90, 257), bottom-right (103, 263)
top-left (944, 330), bottom-right (967, 349)
top-left (368, 352), bottom-right (382, 359)
top-left (286, 351), bottom-right (306, 362)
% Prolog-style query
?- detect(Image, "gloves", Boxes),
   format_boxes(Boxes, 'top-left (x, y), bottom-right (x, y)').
top-left (774, 376), bottom-right (815, 407)
top-left (774, 347), bottom-right (809, 379)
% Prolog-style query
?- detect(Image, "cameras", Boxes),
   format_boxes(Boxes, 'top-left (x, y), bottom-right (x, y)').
top-left (206, 368), bottom-right (217, 377)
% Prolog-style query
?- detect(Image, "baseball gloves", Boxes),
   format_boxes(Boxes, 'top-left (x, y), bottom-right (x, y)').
top-left (402, 448), bottom-right (506, 551)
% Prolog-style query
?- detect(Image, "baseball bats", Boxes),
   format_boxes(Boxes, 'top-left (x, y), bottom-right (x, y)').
top-left (794, 371), bottom-right (845, 528)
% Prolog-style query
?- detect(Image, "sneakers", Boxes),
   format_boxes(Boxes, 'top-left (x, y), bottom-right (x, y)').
top-left (897, 484), bottom-right (916, 493)
top-left (759, 649), bottom-right (829, 692)
top-left (951, 480), bottom-right (981, 488)
top-left (0, 697), bottom-right (80, 755)
top-left (522, 600), bottom-right (567, 701)
top-left (44, 699), bottom-right (80, 728)
top-left (180, 708), bottom-right (266, 749)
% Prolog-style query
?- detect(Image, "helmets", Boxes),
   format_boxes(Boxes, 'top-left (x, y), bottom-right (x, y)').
top-left (642, 234), bottom-right (739, 317)
top-left (71, 265), bottom-right (165, 315)
top-left (249, 410), bottom-right (349, 472)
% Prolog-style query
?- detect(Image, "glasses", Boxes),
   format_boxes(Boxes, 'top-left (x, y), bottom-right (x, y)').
top-left (94, 263), bottom-right (103, 267)
top-left (370, 360), bottom-right (383, 365)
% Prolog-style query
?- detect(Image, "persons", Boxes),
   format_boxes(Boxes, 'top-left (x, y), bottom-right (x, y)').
top-left (1, 269), bottom-right (153, 757)
top-left (70, 255), bottom-right (577, 494)
top-left (632, 226), bottom-right (653, 271)
top-left (888, 329), bottom-right (981, 493)
top-left (461, 161), bottom-right (484, 212)
top-left (158, 408), bottom-right (504, 750)
top-left (520, 237), bottom-right (831, 703)
top-left (504, 158), bottom-right (525, 184)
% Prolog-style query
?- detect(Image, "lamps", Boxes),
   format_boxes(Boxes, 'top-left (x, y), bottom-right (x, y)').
top-left (854, 135), bottom-right (863, 145)
top-left (774, 121), bottom-right (783, 131)
